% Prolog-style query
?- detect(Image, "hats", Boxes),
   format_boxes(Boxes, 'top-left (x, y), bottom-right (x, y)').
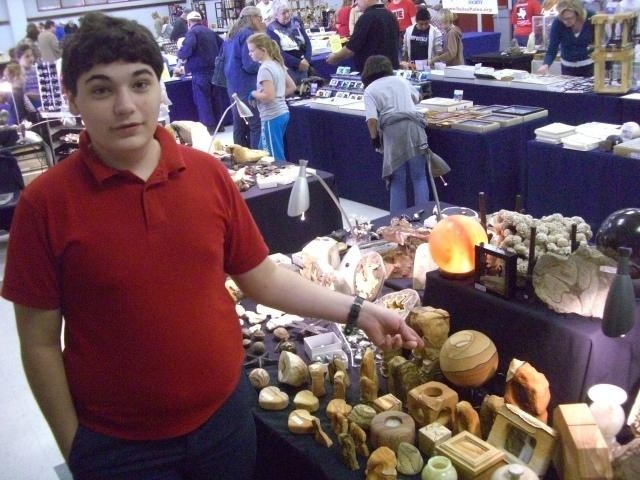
top-left (187, 11), bottom-right (201, 20)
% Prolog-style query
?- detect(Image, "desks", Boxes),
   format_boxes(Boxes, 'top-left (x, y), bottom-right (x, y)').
top-left (164, 30), bottom-right (640, 480)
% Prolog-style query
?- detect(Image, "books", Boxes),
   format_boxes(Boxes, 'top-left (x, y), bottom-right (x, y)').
top-left (418, 65), bottom-right (640, 159)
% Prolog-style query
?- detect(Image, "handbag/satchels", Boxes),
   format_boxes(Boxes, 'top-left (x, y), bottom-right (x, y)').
top-left (211, 56), bottom-right (227, 88)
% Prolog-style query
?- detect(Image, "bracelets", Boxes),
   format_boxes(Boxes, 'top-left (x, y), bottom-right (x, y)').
top-left (343, 292), bottom-right (368, 340)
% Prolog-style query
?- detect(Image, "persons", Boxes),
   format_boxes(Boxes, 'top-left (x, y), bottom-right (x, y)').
top-left (510, 0), bottom-right (639, 78)
top-left (0, 0), bottom-right (429, 230)
top-left (426, 3), bottom-right (463, 66)
top-left (0, 8), bottom-right (425, 479)
